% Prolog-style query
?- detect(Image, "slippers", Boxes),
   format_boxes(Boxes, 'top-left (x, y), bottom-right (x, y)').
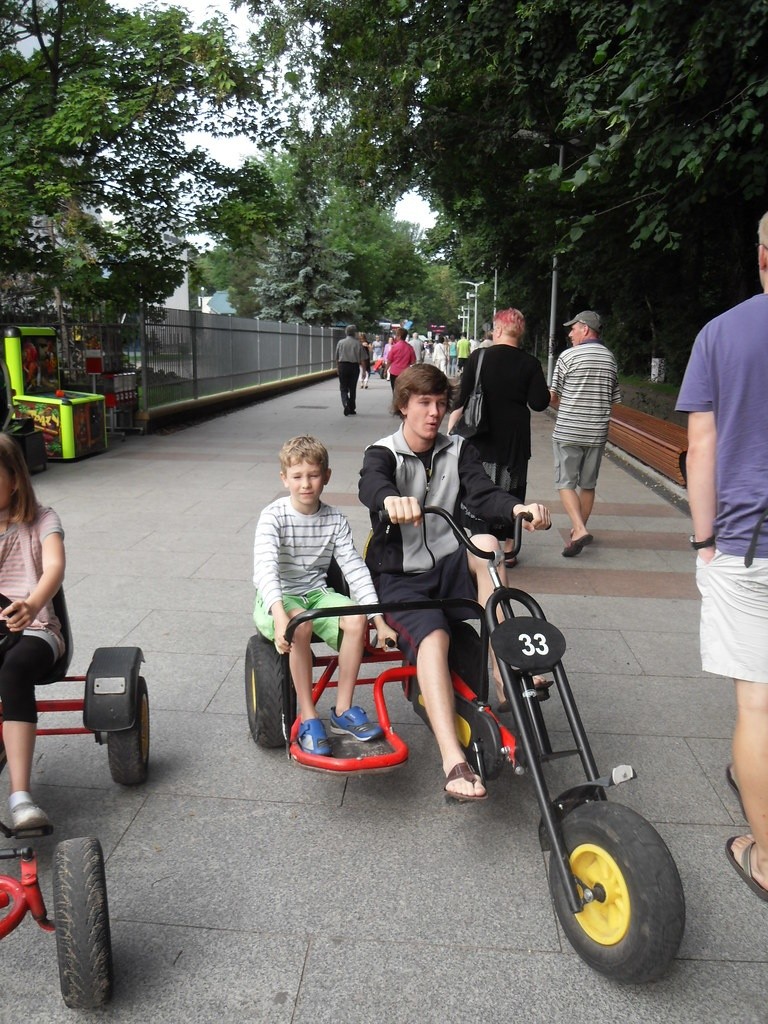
top-left (443, 763), bottom-right (488, 802)
top-left (726, 764), bottom-right (751, 822)
top-left (505, 556), bottom-right (517, 568)
top-left (498, 677), bottom-right (554, 712)
top-left (562, 534), bottom-right (593, 556)
top-left (725, 835), bottom-right (768, 902)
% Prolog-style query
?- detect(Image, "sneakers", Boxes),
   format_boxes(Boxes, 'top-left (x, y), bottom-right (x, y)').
top-left (330, 704), bottom-right (383, 741)
top-left (298, 719), bottom-right (333, 755)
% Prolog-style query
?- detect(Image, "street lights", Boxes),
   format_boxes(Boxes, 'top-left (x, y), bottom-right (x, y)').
top-left (459, 281), bottom-right (484, 341)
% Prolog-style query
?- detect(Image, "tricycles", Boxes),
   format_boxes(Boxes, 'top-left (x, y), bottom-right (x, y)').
top-left (1, 584), bottom-right (150, 1011)
top-left (243, 506), bottom-right (687, 985)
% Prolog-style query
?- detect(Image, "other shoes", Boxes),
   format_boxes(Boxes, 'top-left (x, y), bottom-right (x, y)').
top-left (12, 802), bottom-right (48, 831)
top-left (344, 403), bottom-right (349, 416)
top-left (350, 410), bottom-right (356, 414)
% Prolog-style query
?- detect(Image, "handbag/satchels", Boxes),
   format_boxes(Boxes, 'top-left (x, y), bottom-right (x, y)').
top-left (463, 394), bottom-right (485, 429)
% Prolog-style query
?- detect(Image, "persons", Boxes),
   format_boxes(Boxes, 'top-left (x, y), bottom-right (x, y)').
top-left (458, 307), bottom-right (551, 568)
top-left (359, 328), bottom-right (494, 389)
top-left (382, 328), bottom-right (417, 414)
top-left (336, 325), bottom-right (368, 416)
top-left (0, 432), bottom-right (75, 830)
top-left (358, 363), bottom-right (553, 801)
top-left (673, 211), bottom-right (768, 903)
top-left (550, 311), bottom-right (622, 557)
top-left (252, 434), bottom-right (399, 756)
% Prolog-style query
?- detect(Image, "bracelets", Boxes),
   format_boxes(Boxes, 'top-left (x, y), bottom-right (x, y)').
top-left (689, 534), bottom-right (715, 550)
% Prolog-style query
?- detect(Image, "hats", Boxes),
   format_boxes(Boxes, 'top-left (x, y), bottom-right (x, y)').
top-left (563, 311), bottom-right (603, 334)
